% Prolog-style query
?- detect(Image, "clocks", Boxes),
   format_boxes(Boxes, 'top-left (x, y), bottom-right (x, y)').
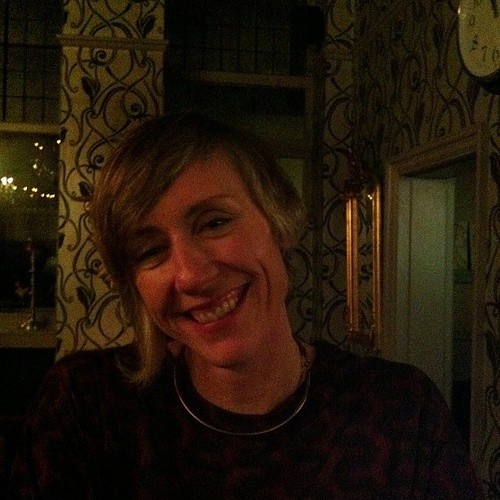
top-left (457, 0), bottom-right (500, 78)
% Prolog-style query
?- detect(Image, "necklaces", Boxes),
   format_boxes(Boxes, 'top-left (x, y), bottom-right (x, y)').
top-left (172, 335), bottom-right (312, 439)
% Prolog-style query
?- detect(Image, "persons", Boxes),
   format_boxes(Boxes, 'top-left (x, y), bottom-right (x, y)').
top-left (0, 109), bottom-right (487, 500)
